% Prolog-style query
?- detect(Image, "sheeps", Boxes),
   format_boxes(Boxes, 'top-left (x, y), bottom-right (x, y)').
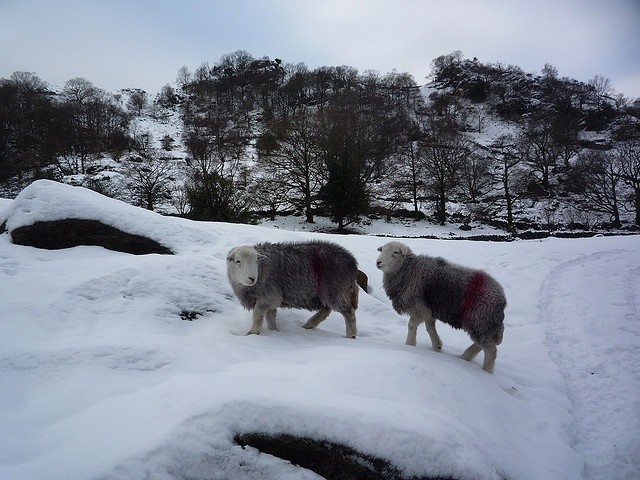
top-left (376, 240), bottom-right (507, 374)
top-left (226, 239), bottom-right (374, 338)
